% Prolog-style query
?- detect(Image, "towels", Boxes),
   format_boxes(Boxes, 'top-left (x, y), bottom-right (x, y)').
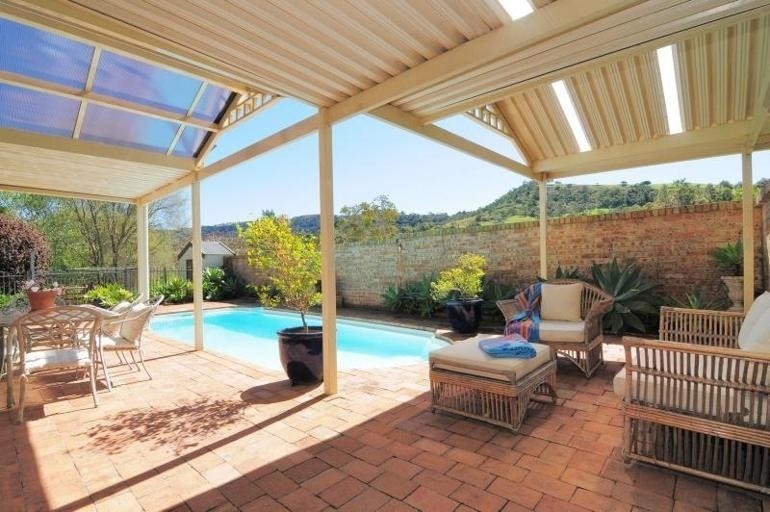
top-left (479, 332), bottom-right (537, 359)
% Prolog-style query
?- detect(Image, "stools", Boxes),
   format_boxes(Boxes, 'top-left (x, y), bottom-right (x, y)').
top-left (429, 333), bottom-right (558, 436)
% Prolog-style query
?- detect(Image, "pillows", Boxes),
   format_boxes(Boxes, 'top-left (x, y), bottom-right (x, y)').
top-left (738, 291), bottom-right (770, 353)
top-left (541, 283), bottom-right (583, 322)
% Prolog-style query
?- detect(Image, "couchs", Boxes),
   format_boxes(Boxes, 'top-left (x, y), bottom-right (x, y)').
top-left (614, 289), bottom-right (770, 496)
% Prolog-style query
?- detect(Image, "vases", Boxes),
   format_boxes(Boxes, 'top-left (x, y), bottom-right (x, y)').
top-left (27, 290), bottom-right (58, 310)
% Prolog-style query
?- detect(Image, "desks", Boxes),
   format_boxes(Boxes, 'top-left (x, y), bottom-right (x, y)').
top-left (0, 304), bottom-right (128, 391)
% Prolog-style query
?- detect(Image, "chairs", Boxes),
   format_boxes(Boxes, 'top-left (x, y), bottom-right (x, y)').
top-left (15, 306), bottom-right (102, 422)
top-left (75, 292), bottom-right (143, 378)
top-left (496, 279), bottom-right (614, 379)
top-left (95, 294), bottom-right (164, 392)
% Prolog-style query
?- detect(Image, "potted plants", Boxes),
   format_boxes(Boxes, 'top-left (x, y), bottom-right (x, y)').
top-left (429, 252), bottom-right (487, 337)
top-left (709, 239), bottom-right (744, 306)
top-left (235, 210), bottom-right (323, 386)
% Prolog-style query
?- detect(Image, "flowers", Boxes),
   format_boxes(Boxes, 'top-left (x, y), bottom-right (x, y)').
top-left (22, 279), bottom-right (63, 295)
